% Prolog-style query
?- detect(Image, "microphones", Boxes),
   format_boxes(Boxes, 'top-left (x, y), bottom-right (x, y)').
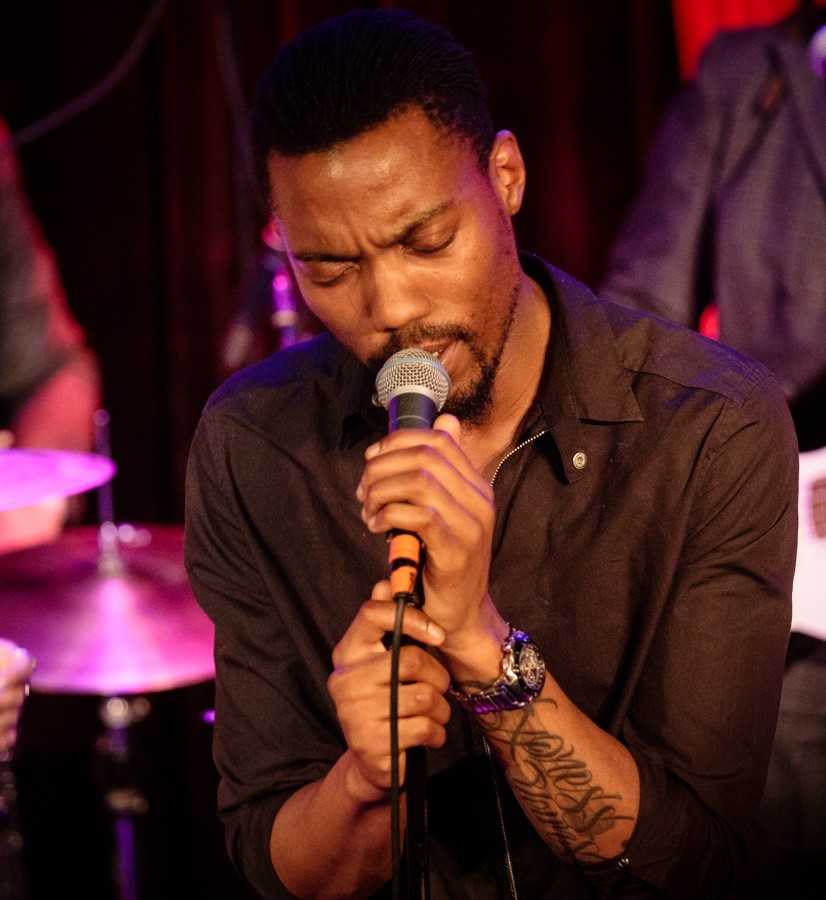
top-left (375, 348), bottom-right (449, 604)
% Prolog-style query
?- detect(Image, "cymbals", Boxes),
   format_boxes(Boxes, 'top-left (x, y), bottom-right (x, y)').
top-left (0, 522), bottom-right (216, 695)
top-left (1, 447), bottom-right (118, 511)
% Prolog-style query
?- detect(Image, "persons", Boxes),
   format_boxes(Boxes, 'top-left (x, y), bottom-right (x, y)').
top-left (597, 0), bottom-right (826, 451)
top-left (0, 110), bottom-right (101, 762)
top-left (184, 10), bottom-right (797, 900)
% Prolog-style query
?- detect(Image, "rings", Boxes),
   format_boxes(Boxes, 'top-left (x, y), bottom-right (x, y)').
top-left (24, 683), bottom-right (30, 695)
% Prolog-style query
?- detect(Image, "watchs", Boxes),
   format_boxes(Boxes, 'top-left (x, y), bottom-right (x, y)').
top-left (448, 622), bottom-right (546, 715)
